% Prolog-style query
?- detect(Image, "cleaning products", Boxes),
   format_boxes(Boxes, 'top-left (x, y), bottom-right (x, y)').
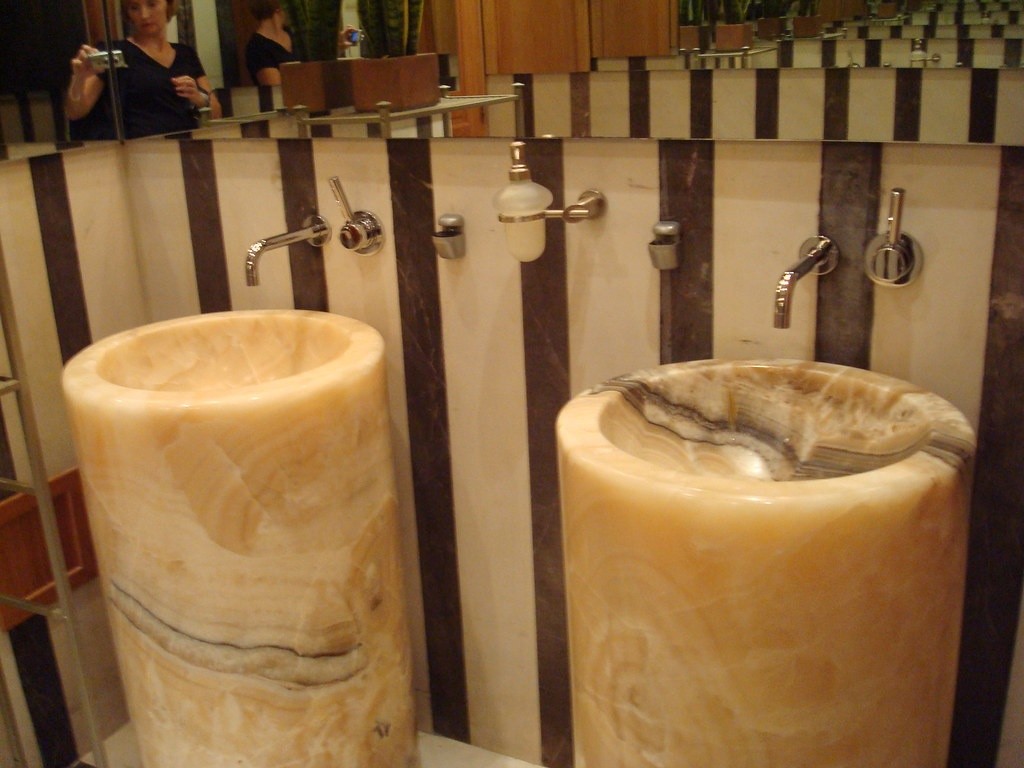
top-left (492, 140), bottom-right (555, 263)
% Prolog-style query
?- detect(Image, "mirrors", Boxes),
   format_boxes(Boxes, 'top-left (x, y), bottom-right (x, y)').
top-left (105, 0), bottom-right (1024, 168)
top-left (0, 0), bottom-right (127, 162)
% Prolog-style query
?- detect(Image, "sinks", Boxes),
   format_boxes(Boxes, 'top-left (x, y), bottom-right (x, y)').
top-left (97, 315), bottom-right (351, 392)
top-left (598, 368), bottom-right (932, 483)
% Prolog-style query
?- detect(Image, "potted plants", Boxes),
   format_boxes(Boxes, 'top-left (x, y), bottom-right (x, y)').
top-left (349, 0), bottom-right (441, 117)
top-left (879, 0), bottom-right (897, 19)
top-left (279, 0), bottom-right (353, 113)
top-left (679, 0), bottom-right (712, 52)
top-left (715, 0), bottom-right (753, 52)
top-left (792, 0), bottom-right (823, 39)
top-left (757, 0), bottom-right (786, 38)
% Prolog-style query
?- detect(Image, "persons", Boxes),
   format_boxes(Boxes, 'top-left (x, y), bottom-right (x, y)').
top-left (65, 0), bottom-right (212, 143)
top-left (242, 0), bottom-right (358, 88)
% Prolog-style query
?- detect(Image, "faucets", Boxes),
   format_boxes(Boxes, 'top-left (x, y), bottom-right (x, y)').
top-left (245, 214), bottom-right (332, 286)
top-left (772, 236), bottom-right (839, 329)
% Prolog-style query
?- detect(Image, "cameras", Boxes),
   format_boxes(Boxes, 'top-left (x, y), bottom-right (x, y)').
top-left (351, 29), bottom-right (364, 42)
top-left (88, 50), bottom-right (126, 71)
